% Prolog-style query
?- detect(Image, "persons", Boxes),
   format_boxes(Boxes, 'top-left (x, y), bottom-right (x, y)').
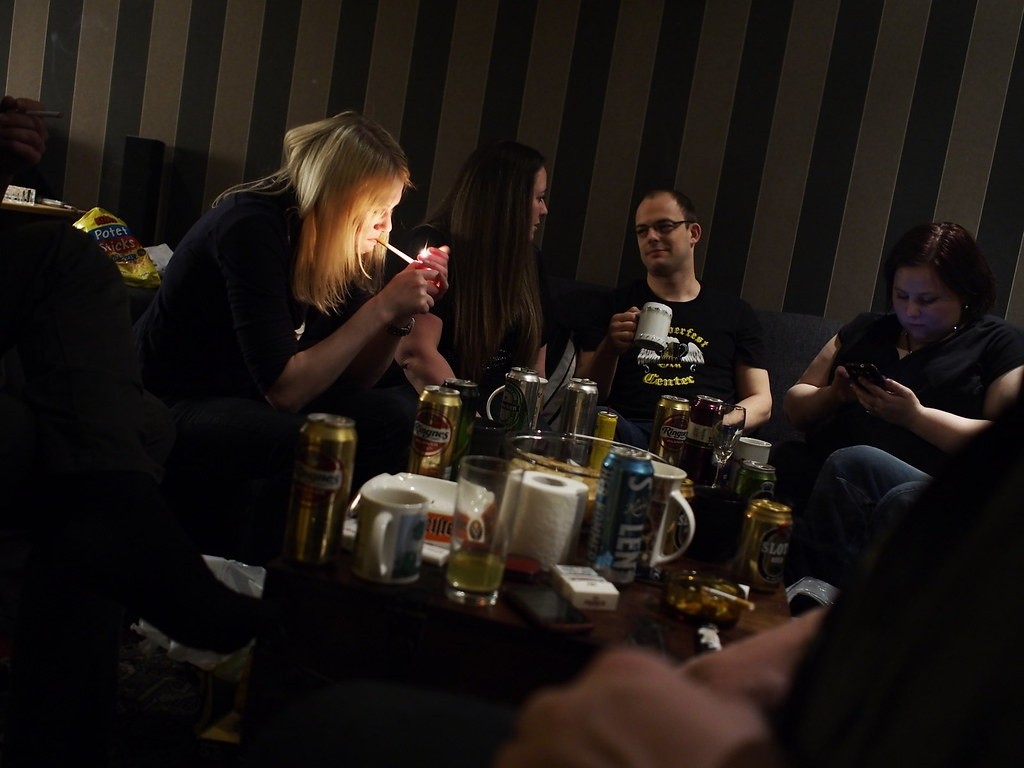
top-left (392, 144), bottom-right (555, 406)
top-left (766, 223), bottom-right (1024, 584)
top-left (0, 99), bottom-right (254, 768)
top-left (571, 189), bottom-right (777, 476)
top-left (132, 110), bottom-right (452, 626)
top-left (496, 383), bottom-right (1024, 767)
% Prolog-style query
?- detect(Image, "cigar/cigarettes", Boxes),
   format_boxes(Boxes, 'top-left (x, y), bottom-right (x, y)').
top-left (375, 238), bottom-right (415, 265)
top-left (21, 110), bottom-right (63, 118)
top-left (701, 586), bottom-right (756, 610)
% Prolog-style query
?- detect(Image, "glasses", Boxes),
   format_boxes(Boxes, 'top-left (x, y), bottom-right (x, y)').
top-left (632, 219), bottom-right (696, 239)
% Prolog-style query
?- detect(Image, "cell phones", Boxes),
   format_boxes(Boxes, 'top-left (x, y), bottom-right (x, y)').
top-left (501, 584), bottom-right (594, 634)
top-left (846, 362), bottom-right (888, 394)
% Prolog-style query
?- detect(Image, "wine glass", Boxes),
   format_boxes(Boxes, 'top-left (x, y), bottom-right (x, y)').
top-left (709, 403), bottom-right (746, 488)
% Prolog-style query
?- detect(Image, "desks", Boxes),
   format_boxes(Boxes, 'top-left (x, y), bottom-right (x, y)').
top-left (248, 546), bottom-right (793, 701)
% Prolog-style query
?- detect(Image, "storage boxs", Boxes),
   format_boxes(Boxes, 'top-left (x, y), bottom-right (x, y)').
top-left (549, 563), bottom-right (620, 610)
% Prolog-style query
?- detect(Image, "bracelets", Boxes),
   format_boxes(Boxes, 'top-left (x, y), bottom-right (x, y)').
top-left (384, 316), bottom-right (415, 338)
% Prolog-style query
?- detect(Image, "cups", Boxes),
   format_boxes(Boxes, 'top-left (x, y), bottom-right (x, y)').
top-left (633, 302), bottom-right (672, 352)
top-left (354, 487), bottom-right (428, 583)
top-left (731, 437), bottom-right (772, 488)
top-left (487, 373), bottom-right (548, 431)
top-left (444, 456), bottom-right (524, 606)
top-left (650, 460), bottom-right (695, 570)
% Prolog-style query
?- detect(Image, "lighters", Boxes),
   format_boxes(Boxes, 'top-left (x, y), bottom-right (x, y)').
top-left (692, 617), bottom-right (720, 654)
top-left (651, 563), bottom-right (741, 597)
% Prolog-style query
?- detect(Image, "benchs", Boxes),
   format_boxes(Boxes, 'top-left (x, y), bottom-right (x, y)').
top-left (537, 276), bottom-right (845, 477)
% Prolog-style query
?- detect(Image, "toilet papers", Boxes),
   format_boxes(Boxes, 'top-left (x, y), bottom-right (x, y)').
top-left (493, 468), bottom-right (590, 572)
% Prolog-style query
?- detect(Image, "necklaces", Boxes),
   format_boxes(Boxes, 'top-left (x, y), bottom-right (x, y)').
top-left (903, 330), bottom-right (913, 353)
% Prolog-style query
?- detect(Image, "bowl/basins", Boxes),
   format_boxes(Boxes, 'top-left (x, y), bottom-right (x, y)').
top-left (488, 431), bottom-right (665, 563)
